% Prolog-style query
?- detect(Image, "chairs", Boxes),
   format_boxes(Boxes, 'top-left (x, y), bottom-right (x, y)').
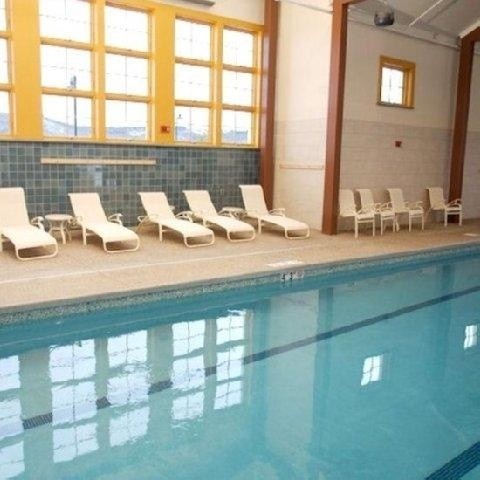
top-left (181, 189), bottom-right (255, 242)
top-left (1, 186), bottom-right (58, 261)
top-left (68, 192), bottom-right (140, 254)
top-left (239, 184), bottom-right (309, 240)
top-left (338, 186), bottom-right (463, 241)
top-left (134, 192), bottom-right (215, 248)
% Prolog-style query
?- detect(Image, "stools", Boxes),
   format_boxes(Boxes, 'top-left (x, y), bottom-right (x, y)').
top-left (219, 206), bottom-right (246, 221)
top-left (44, 213), bottom-right (72, 245)
